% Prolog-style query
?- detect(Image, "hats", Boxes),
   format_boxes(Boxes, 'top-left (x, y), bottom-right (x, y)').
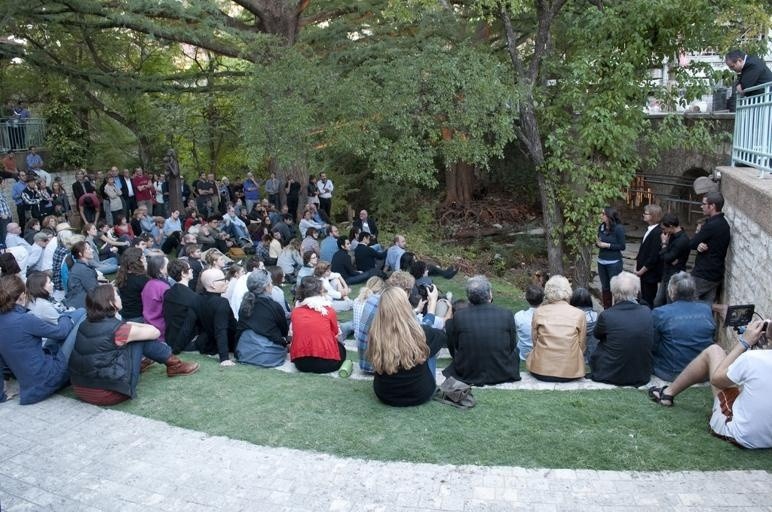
top-left (24, 176), bottom-right (38, 183)
top-left (33, 232), bottom-right (50, 241)
top-left (56, 223), bottom-right (77, 232)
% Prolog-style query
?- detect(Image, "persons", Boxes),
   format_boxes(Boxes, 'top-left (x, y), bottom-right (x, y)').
top-left (725, 50), bottom-right (772, 171)
top-left (589, 271), bottom-right (654, 388)
top-left (69, 284), bottom-right (200, 404)
top-left (596, 206), bottom-right (626, 308)
top-left (571, 288), bottom-right (597, 363)
top-left (525, 274), bottom-right (587, 383)
top-left (652, 271), bottom-right (716, 382)
top-left (4, 99), bottom-right (17, 152)
top-left (367, 286), bottom-right (447, 406)
top-left (649, 319), bottom-right (772, 449)
top-left (633, 204), bottom-right (664, 309)
top-left (359, 271), bottom-right (438, 373)
top-left (15, 101), bottom-right (30, 151)
top-left (514, 285), bottom-right (544, 361)
top-left (688, 191), bottom-right (730, 304)
top-left (446, 274), bottom-right (522, 386)
top-left (653, 213), bottom-right (691, 308)
top-left (1, 276), bottom-right (86, 404)
top-left (1, 147), bottom-right (458, 367)
top-left (291, 275), bottom-right (346, 373)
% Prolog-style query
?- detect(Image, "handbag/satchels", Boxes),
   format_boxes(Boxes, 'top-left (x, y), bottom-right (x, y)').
top-left (0, 252), bottom-right (21, 276)
top-left (432, 375), bottom-right (477, 408)
top-left (198, 232), bottom-right (215, 244)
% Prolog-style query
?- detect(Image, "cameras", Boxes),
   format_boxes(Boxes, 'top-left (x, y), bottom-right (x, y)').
top-left (723, 304), bottom-right (756, 328)
top-left (418, 282), bottom-right (433, 296)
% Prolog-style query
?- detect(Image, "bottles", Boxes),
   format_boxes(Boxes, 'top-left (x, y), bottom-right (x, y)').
top-left (338, 358), bottom-right (353, 378)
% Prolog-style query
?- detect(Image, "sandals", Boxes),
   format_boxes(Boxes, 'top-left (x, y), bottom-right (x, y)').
top-left (648, 385), bottom-right (674, 407)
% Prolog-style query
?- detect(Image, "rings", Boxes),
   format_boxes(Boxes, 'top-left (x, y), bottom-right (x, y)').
top-left (701, 246), bottom-right (702, 248)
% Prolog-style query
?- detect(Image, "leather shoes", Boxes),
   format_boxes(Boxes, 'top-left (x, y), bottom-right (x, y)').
top-left (140, 358), bottom-right (155, 371)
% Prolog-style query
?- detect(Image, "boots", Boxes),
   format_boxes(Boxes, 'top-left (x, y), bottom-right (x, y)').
top-left (165, 354), bottom-right (199, 376)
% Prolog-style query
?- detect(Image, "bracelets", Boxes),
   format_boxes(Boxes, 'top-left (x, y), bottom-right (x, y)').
top-left (738, 337), bottom-right (749, 350)
top-left (662, 243), bottom-right (668, 245)
